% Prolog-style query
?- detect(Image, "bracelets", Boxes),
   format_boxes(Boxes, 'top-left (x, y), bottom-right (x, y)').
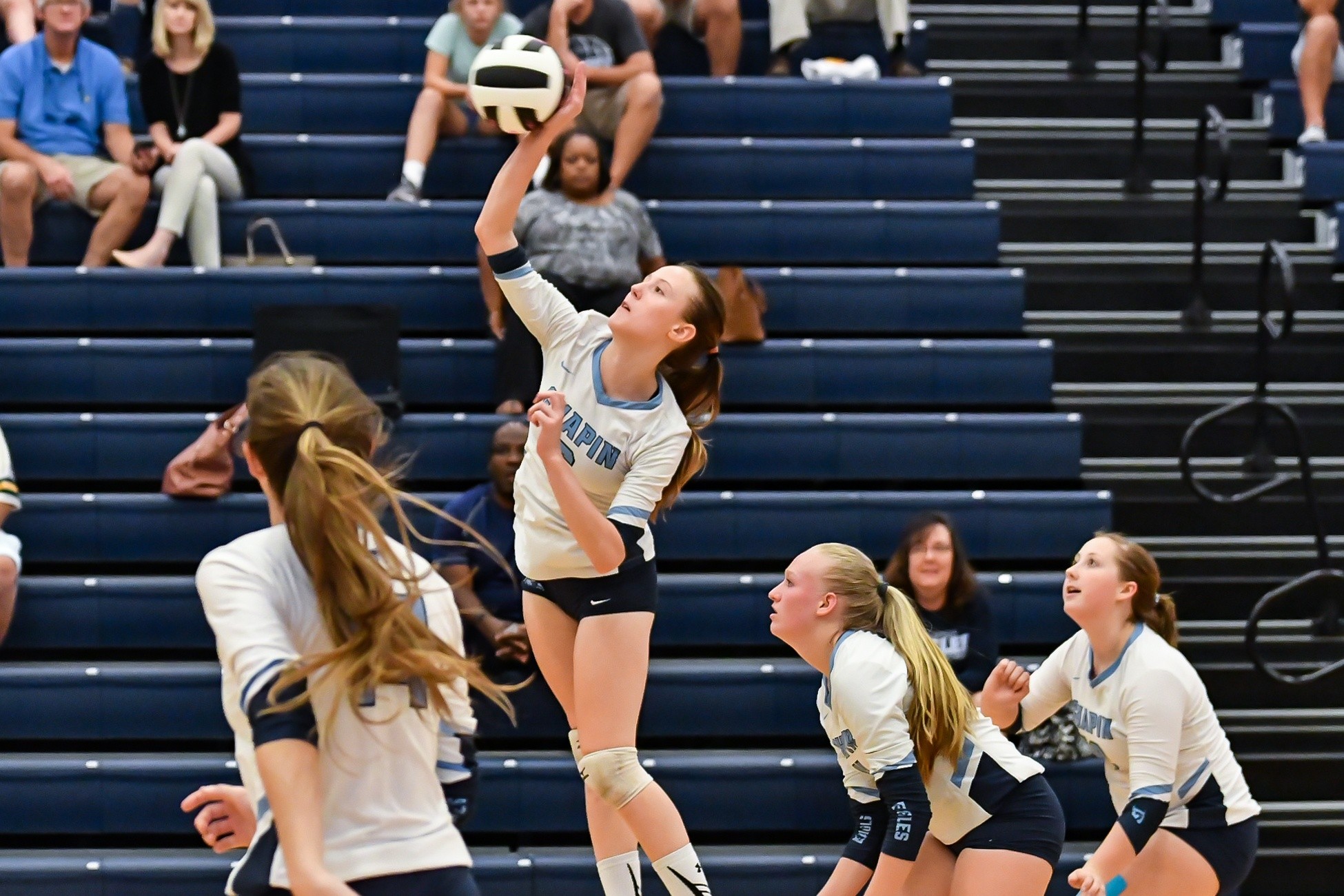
top-left (472, 611), bottom-right (491, 628)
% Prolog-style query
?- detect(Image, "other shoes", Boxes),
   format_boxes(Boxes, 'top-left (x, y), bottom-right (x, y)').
top-left (1298, 126), bottom-right (1326, 143)
top-left (112, 249), bottom-right (165, 269)
top-left (768, 57), bottom-right (791, 75)
top-left (891, 48), bottom-right (922, 75)
top-left (386, 185), bottom-right (419, 204)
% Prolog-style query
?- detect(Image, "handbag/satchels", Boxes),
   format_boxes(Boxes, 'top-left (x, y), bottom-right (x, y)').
top-left (800, 53), bottom-right (881, 83)
top-left (161, 400), bottom-right (250, 497)
top-left (225, 218), bottom-right (317, 268)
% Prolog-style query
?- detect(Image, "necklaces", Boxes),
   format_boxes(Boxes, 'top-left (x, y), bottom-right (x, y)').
top-left (172, 57), bottom-right (197, 138)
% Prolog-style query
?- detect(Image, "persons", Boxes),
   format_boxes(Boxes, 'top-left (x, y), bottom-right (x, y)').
top-left (979, 529), bottom-right (1262, 895)
top-left (386, 0), bottom-right (744, 206)
top-left (1290, 0), bottom-right (1344, 145)
top-left (767, 1), bottom-right (921, 79)
top-left (477, 127), bottom-right (668, 416)
top-left (0, 0), bottom-right (151, 267)
top-left (0, 428), bottom-right (22, 641)
top-left (768, 542), bottom-right (1066, 895)
top-left (179, 352), bottom-right (534, 896)
top-left (474, 62), bottom-right (726, 896)
top-left (883, 511), bottom-right (1000, 710)
top-left (432, 420), bottom-right (531, 825)
top-left (110, 0), bottom-right (251, 270)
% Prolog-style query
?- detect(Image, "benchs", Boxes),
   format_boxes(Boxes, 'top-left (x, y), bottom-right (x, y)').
top-left (0, 0), bottom-right (1125, 896)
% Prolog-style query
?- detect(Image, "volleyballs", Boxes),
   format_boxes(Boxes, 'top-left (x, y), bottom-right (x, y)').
top-left (467, 34), bottom-right (565, 135)
